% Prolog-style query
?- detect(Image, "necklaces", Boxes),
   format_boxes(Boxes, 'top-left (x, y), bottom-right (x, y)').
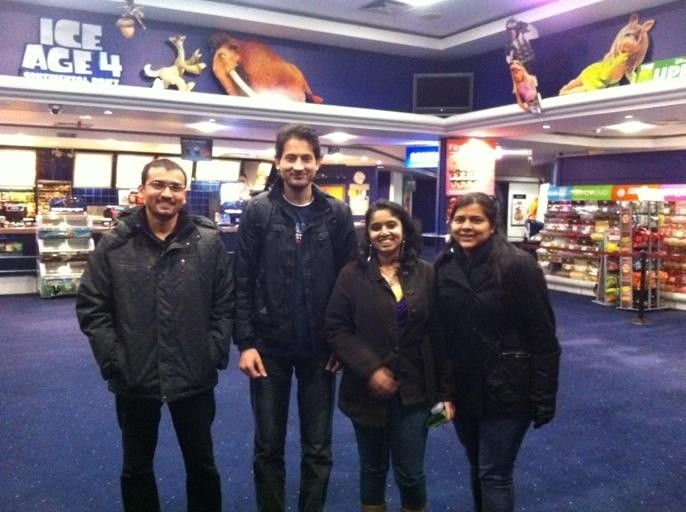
top-left (381, 269), bottom-right (396, 288)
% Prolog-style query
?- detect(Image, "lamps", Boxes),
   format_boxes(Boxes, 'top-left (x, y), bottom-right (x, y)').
top-left (117, 3), bottom-right (147, 39)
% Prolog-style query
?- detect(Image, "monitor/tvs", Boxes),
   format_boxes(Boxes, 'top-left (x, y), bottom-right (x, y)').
top-left (412, 73), bottom-right (474, 117)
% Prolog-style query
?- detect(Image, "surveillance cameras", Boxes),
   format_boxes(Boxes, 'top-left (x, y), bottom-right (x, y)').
top-left (48, 104), bottom-right (63, 114)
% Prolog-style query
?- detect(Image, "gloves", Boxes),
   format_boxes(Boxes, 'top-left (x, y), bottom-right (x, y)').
top-left (532, 412), bottom-right (552, 428)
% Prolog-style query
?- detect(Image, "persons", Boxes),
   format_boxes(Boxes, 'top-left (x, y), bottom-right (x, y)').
top-left (76, 158), bottom-right (234, 512)
top-left (504, 18), bottom-right (539, 74)
top-left (323, 198), bottom-right (435, 512)
top-left (434, 192), bottom-right (562, 512)
top-left (233, 123), bottom-right (360, 512)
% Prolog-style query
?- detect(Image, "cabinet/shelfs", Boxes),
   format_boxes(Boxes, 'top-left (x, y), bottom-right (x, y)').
top-left (538, 200), bottom-right (685, 309)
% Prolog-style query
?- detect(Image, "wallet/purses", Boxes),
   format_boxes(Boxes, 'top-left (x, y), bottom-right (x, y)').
top-left (426, 409), bottom-right (446, 428)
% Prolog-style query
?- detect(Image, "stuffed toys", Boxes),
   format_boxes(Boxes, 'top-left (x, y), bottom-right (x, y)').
top-left (509, 61), bottom-right (542, 113)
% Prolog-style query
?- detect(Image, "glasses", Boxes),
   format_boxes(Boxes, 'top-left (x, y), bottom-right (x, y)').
top-left (146, 181), bottom-right (184, 192)
